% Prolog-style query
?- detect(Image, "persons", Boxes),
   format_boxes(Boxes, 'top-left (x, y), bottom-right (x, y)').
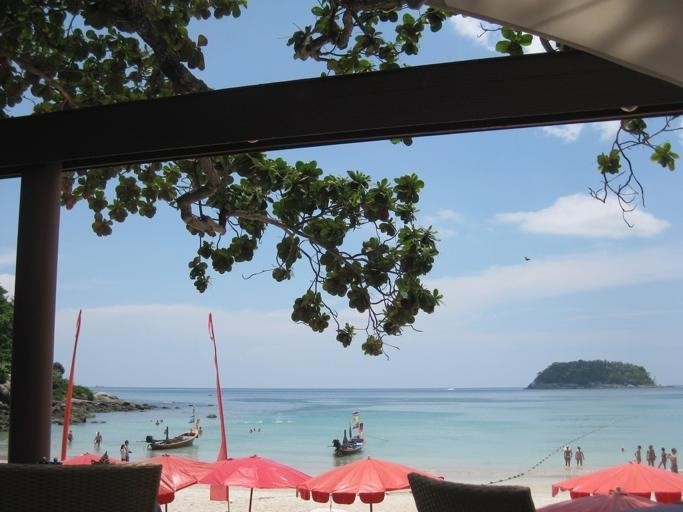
top-left (67, 419), bottom-right (260, 462)
top-left (563, 444), bottom-right (572, 466)
top-left (620, 443), bottom-right (678, 473)
top-left (36, 455), bottom-right (48, 464)
top-left (574, 445), bottom-right (584, 466)
top-left (50, 457), bottom-right (60, 464)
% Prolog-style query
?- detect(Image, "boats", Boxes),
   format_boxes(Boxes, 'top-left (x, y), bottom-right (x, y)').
top-left (145, 425), bottom-right (197, 450)
top-left (331, 434), bottom-right (363, 456)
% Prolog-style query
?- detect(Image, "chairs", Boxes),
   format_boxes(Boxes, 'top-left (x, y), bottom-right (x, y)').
top-left (407, 471), bottom-right (536, 512)
top-left (0, 462), bottom-right (163, 512)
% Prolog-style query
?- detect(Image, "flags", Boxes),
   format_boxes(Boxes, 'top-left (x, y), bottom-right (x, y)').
top-left (351, 410), bottom-right (360, 429)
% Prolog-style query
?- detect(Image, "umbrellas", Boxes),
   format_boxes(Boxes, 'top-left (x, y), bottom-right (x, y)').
top-left (535, 490), bottom-right (661, 512)
top-left (122, 452), bottom-right (215, 512)
top-left (196, 454), bottom-right (314, 512)
top-left (553, 460), bottom-right (682, 503)
top-left (60, 453), bottom-right (118, 465)
top-left (296, 456), bottom-right (444, 511)
